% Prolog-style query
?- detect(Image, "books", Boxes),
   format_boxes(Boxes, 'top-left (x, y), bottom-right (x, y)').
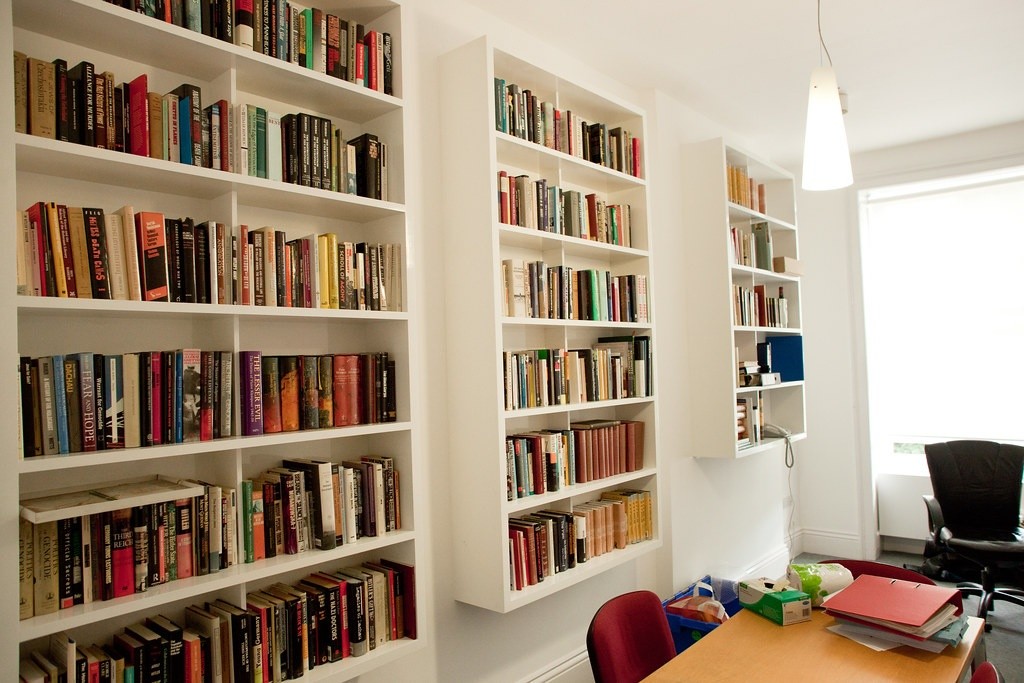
top-left (12, 0), bottom-right (406, 318)
top-left (496, 78), bottom-right (655, 593)
top-left (726, 165), bottom-right (805, 451)
top-left (19, 349), bottom-right (418, 683)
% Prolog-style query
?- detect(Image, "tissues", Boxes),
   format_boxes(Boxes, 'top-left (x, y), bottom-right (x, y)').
top-left (737, 577), bottom-right (814, 627)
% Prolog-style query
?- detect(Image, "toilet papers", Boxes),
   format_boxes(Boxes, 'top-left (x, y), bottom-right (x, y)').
top-left (785, 562), bottom-right (854, 608)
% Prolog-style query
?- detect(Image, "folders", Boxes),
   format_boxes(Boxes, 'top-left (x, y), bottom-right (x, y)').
top-left (766, 336), bottom-right (803, 381)
top-left (819, 573), bottom-right (963, 642)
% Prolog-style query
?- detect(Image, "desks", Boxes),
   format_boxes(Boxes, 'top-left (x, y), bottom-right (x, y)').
top-left (638, 606), bottom-right (987, 683)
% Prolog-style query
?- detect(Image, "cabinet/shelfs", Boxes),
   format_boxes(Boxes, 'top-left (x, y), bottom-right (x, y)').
top-left (423, 33), bottom-right (663, 615)
top-left (0, 0), bottom-right (437, 683)
top-left (677, 135), bottom-right (808, 459)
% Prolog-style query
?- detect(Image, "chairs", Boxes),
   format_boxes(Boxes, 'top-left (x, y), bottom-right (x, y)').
top-left (586, 590), bottom-right (677, 683)
top-left (817, 559), bottom-right (938, 586)
top-left (921, 439), bottom-right (1024, 633)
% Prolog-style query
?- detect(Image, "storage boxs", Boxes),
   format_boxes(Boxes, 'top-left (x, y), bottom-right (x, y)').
top-left (739, 576), bottom-right (812, 627)
top-left (773, 255), bottom-right (804, 277)
top-left (661, 575), bottom-right (744, 655)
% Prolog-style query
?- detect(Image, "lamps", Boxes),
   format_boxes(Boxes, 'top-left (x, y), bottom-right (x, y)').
top-left (801, 39), bottom-right (853, 192)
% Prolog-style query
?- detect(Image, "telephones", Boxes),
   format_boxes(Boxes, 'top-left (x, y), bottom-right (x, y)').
top-left (764, 421), bottom-right (792, 438)
